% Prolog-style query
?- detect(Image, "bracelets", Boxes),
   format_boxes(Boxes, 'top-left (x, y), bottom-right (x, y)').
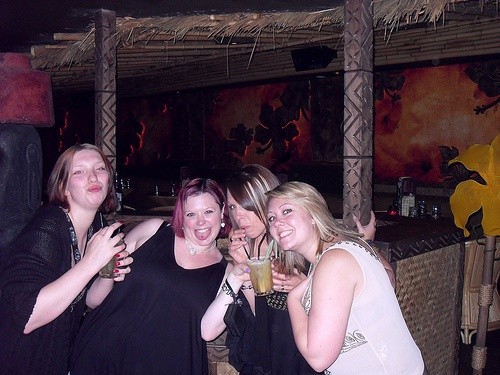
top-left (365, 240), bottom-right (376, 248)
top-left (221, 279), bottom-right (242, 305)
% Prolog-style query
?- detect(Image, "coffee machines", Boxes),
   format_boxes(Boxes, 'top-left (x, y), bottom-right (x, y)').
top-left (397, 176), bottom-right (416, 217)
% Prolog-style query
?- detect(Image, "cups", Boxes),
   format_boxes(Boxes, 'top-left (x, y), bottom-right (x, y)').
top-left (417, 200), bottom-right (426, 216)
top-left (97, 239), bottom-right (125, 279)
top-left (431, 201), bottom-right (441, 216)
top-left (246, 256), bottom-right (274, 297)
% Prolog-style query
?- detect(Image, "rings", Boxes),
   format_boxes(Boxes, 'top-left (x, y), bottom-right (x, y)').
top-left (281, 285), bottom-right (285, 291)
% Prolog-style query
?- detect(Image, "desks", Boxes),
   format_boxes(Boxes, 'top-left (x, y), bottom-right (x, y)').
top-left (332, 208), bottom-right (437, 245)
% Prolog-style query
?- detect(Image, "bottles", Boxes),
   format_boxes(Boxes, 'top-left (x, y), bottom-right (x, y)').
top-left (114, 172), bottom-right (131, 211)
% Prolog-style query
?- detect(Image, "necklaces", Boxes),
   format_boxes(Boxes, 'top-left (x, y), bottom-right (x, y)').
top-left (183, 240), bottom-right (217, 256)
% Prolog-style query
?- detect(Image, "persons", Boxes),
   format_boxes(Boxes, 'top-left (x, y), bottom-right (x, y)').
top-left (202, 164), bottom-right (323, 374)
top-left (70, 178), bottom-right (233, 375)
top-left (1, 143), bottom-right (134, 375)
top-left (264, 180), bottom-right (425, 375)
